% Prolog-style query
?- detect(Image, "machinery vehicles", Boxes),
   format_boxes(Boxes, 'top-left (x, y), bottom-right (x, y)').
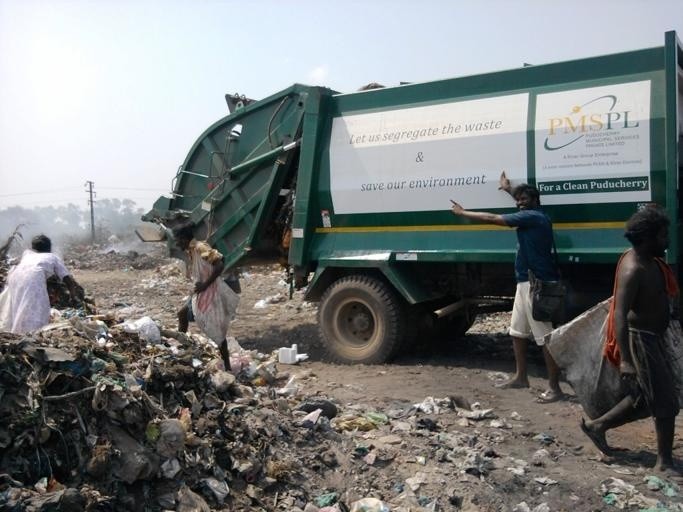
top-left (142, 31), bottom-right (682, 366)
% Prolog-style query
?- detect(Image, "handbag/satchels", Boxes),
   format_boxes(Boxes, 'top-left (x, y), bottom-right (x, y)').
top-left (527, 278), bottom-right (569, 324)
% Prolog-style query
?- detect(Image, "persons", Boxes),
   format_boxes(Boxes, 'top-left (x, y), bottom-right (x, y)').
top-left (0, 235), bottom-right (80, 335)
top-left (450, 171), bottom-right (563, 404)
top-left (172, 222), bottom-right (231, 371)
top-left (580, 205), bottom-right (683, 485)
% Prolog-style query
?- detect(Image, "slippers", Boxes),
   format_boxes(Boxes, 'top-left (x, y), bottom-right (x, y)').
top-left (534, 387), bottom-right (565, 404)
top-left (645, 464), bottom-right (683, 485)
top-left (493, 378), bottom-right (530, 389)
top-left (577, 415), bottom-right (613, 457)
